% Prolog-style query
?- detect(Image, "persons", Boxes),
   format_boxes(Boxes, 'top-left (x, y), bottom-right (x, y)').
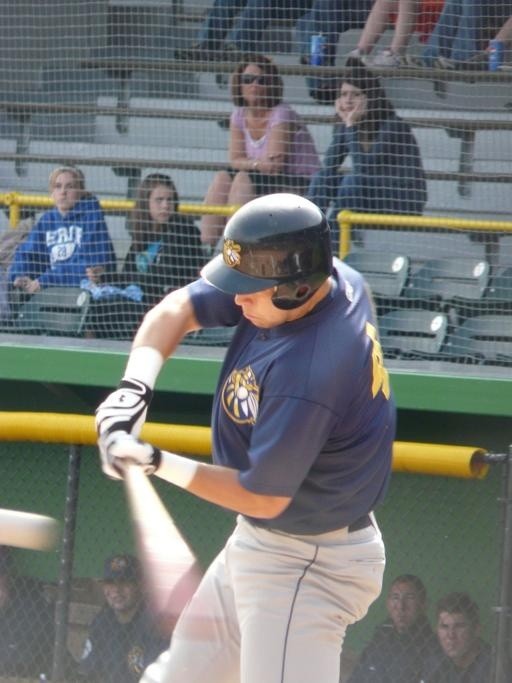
top-left (346, 0), bottom-right (422, 68)
top-left (198, 50), bottom-right (326, 250)
top-left (90, 189), bottom-right (397, 683)
top-left (305, 68), bottom-right (427, 258)
top-left (172, 0), bottom-right (284, 56)
top-left (6, 163), bottom-right (117, 336)
top-left (420, 587), bottom-right (500, 683)
top-left (347, 571), bottom-right (437, 681)
top-left (73, 550), bottom-right (174, 681)
top-left (476, 15), bottom-right (512, 70)
top-left (406, 0), bottom-right (489, 70)
top-left (84, 173), bottom-right (205, 334)
top-left (295, 1), bottom-right (370, 64)
top-left (0, 545), bottom-right (56, 681)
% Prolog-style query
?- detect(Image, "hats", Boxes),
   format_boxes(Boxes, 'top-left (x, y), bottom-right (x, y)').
top-left (97, 554), bottom-right (140, 583)
top-left (199, 190), bottom-right (332, 311)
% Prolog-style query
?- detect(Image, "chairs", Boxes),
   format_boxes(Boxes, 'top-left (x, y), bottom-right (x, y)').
top-left (0, 23), bottom-right (512, 366)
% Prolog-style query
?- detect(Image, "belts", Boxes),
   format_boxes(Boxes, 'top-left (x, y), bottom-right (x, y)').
top-left (349, 514), bottom-right (373, 533)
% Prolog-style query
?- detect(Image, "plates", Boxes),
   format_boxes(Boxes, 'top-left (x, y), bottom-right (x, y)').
top-left (497, 65), bottom-right (512, 72)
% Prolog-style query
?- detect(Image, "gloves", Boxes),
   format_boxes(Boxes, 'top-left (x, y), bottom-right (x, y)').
top-left (95, 377), bottom-right (154, 440)
top-left (97, 429), bottom-right (160, 481)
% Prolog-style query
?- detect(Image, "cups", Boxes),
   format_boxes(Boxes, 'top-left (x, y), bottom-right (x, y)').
top-left (310, 34), bottom-right (326, 65)
top-left (487, 41), bottom-right (500, 68)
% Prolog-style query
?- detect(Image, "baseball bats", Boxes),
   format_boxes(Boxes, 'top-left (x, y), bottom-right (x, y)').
top-left (124, 458), bottom-right (196, 608)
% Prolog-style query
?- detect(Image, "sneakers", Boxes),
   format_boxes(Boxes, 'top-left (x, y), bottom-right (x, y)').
top-left (174, 43), bottom-right (242, 61)
top-left (346, 49), bottom-right (454, 69)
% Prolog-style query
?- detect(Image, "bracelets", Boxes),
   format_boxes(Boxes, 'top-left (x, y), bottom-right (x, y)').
top-left (119, 347), bottom-right (163, 391)
top-left (155, 443), bottom-right (199, 491)
